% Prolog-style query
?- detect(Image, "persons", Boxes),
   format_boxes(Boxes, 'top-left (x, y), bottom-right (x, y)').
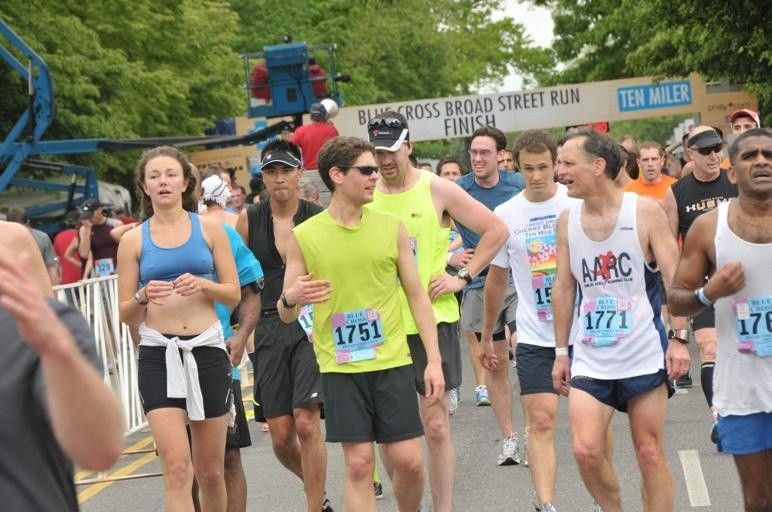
top-left (250, 60), bottom-right (271, 103)
top-left (306, 55), bottom-right (330, 98)
top-left (0, 104), bottom-right (772, 512)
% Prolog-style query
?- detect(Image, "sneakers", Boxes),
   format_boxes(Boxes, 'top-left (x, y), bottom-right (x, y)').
top-left (498, 438), bottom-right (520, 466)
top-left (475, 385), bottom-right (490, 407)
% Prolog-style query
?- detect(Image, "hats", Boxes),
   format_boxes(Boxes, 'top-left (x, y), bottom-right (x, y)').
top-left (81, 199), bottom-right (98, 219)
top-left (731, 109), bottom-right (760, 127)
top-left (259, 150), bottom-right (302, 169)
top-left (687, 129), bottom-right (722, 149)
top-left (368, 111), bottom-right (410, 153)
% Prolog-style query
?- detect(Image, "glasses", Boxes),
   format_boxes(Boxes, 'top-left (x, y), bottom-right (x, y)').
top-left (338, 165), bottom-right (379, 175)
top-left (691, 147), bottom-right (721, 156)
top-left (468, 150), bottom-right (499, 158)
top-left (368, 118), bottom-right (402, 129)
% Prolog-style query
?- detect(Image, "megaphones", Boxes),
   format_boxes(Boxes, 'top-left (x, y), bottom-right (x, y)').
top-left (319, 98), bottom-right (340, 119)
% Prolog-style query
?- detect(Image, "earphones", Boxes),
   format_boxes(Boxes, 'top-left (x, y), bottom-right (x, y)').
top-left (407, 142), bottom-right (410, 148)
